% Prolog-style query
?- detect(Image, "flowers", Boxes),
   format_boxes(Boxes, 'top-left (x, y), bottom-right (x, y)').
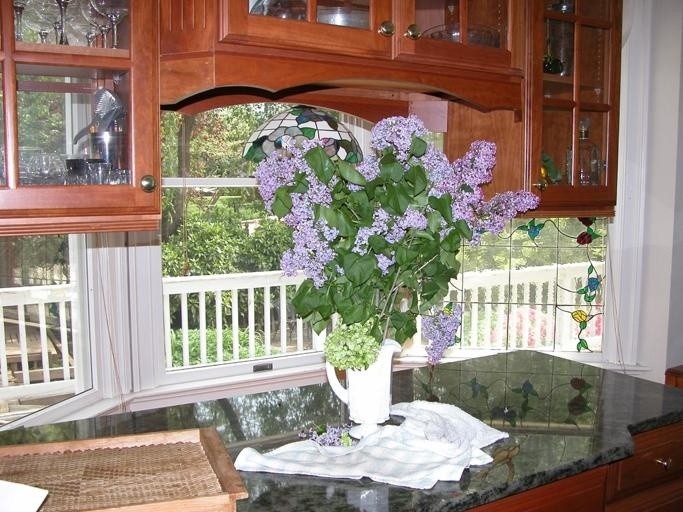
top-left (251, 113), bottom-right (543, 372)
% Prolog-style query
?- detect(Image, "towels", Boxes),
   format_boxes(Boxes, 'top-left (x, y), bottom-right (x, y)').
top-left (233, 398), bottom-right (509, 490)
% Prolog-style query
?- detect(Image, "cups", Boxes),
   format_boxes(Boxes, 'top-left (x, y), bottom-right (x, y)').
top-left (7, 145), bottom-right (131, 185)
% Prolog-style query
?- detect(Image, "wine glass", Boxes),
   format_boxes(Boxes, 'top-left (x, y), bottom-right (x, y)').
top-left (13, 0), bottom-right (130, 48)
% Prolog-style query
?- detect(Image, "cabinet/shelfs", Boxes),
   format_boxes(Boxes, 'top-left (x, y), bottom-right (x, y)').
top-left (530, 0), bottom-right (623, 216)
top-left (162, 1), bottom-right (528, 81)
top-left (0, 0), bottom-right (160, 237)
top-left (469, 424), bottom-right (682, 512)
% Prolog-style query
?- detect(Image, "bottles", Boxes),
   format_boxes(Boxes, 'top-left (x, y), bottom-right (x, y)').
top-left (324, 338), bottom-right (404, 442)
top-left (550, 4), bottom-right (577, 76)
top-left (563, 117), bottom-right (603, 183)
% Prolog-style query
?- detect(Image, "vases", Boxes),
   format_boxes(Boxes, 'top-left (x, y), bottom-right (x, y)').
top-left (325, 335), bottom-right (403, 426)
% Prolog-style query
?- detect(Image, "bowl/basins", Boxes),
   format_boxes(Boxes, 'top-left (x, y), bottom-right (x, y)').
top-left (420, 22), bottom-right (502, 48)
